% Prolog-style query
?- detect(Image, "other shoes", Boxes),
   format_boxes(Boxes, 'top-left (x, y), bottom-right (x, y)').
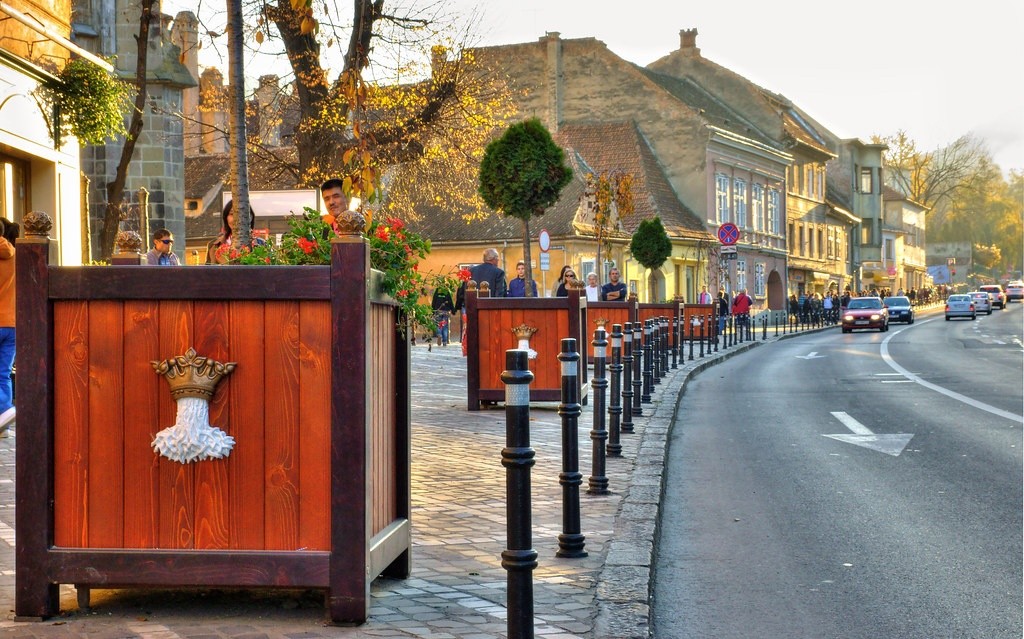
top-left (443, 342), bottom-right (447, 347)
top-left (437, 335), bottom-right (441, 346)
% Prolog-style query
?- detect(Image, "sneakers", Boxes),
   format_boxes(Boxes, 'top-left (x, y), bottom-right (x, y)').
top-left (0, 406), bottom-right (16, 438)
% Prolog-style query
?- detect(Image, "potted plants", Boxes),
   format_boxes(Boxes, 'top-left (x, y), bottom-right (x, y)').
top-left (628, 217), bottom-right (685, 352)
top-left (582, 173), bottom-right (636, 367)
top-left (466, 117), bottom-right (589, 411)
top-left (686, 236), bottom-right (722, 342)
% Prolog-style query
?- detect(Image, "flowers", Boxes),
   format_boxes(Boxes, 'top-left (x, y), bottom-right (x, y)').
top-left (195, 174), bottom-right (470, 351)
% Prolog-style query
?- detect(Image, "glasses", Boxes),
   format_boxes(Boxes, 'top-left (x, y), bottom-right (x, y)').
top-left (565, 275), bottom-right (574, 277)
top-left (158, 240), bottom-right (174, 244)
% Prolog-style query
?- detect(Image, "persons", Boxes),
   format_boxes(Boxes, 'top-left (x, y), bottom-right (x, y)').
top-left (320, 179), bottom-right (348, 238)
top-left (205, 199), bottom-right (266, 265)
top-left (147, 228), bottom-right (180, 266)
top-left (697, 286), bottom-right (753, 342)
top-left (786, 283), bottom-right (956, 325)
top-left (432, 282), bottom-right (469, 347)
top-left (470, 248), bottom-right (507, 297)
top-left (551, 265), bottom-right (627, 302)
top-left (508, 262), bottom-right (538, 297)
top-left (0, 216), bottom-right (20, 438)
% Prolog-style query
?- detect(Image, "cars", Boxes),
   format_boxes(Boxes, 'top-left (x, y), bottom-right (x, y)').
top-left (839, 298), bottom-right (890, 333)
top-left (945, 295), bottom-right (977, 321)
top-left (967, 292), bottom-right (993, 315)
top-left (882, 296), bottom-right (915, 325)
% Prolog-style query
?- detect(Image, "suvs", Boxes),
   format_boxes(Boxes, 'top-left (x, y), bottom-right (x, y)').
top-left (1004, 283), bottom-right (1024, 302)
top-left (979, 285), bottom-right (1007, 309)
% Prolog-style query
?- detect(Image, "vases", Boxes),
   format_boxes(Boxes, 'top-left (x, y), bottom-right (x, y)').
top-left (15, 209), bottom-right (411, 622)
